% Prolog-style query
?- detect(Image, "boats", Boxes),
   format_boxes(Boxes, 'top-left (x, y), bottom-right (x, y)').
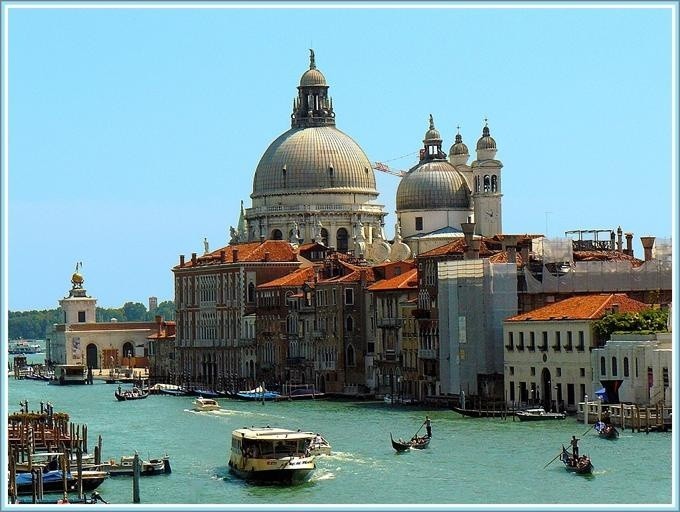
top-left (598, 425), bottom-right (618, 439)
top-left (114, 389), bottom-right (149, 400)
top-left (9, 342), bottom-right (42, 354)
top-left (15, 454), bottom-right (164, 496)
top-left (389, 432), bottom-right (431, 452)
top-left (229, 425), bottom-right (331, 483)
top-left (193, 387), bottom-right (281, 400)
top-left (48, 365), bottom-right (89, 386)
top-left (562, 445), bottom-right (591, 472)
top-left (517, 412), bottom-right (564, 421)
top-left (193, 396), bottom-right (220, 411)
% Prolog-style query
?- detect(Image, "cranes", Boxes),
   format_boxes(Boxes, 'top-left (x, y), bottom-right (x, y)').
top-left (371, 148), bottom-right (427, 178)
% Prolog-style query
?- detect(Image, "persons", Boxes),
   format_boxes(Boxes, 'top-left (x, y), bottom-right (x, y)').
top-left (118, 385), bottom-right (122, 395)
top-left (605, 408), bottom-right (611, 423)
top-left (425, 416), bottom-right (431, 437)
top-left (91, 489), bottom-right (107, 504)
top-left (571, 436), bottom-right (580, 454)
top-left (133, 385), bottom-right (145, 396)
